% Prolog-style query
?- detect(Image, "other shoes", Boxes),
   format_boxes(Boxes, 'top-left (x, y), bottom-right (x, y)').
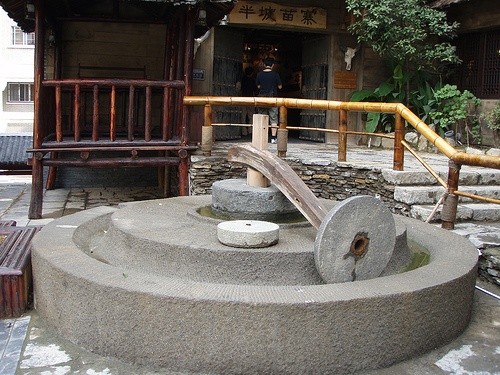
top-left (271, 139), bottom-right (278, 144)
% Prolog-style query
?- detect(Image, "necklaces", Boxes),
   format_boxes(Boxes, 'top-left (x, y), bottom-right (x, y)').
top-left (264, 68), bottom-right (272, 69)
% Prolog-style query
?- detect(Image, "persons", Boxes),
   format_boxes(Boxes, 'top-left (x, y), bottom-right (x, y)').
top-left (258, 58), bottom-right (282, 142)
top-left (235, 66), bottom-right (260, 140)
